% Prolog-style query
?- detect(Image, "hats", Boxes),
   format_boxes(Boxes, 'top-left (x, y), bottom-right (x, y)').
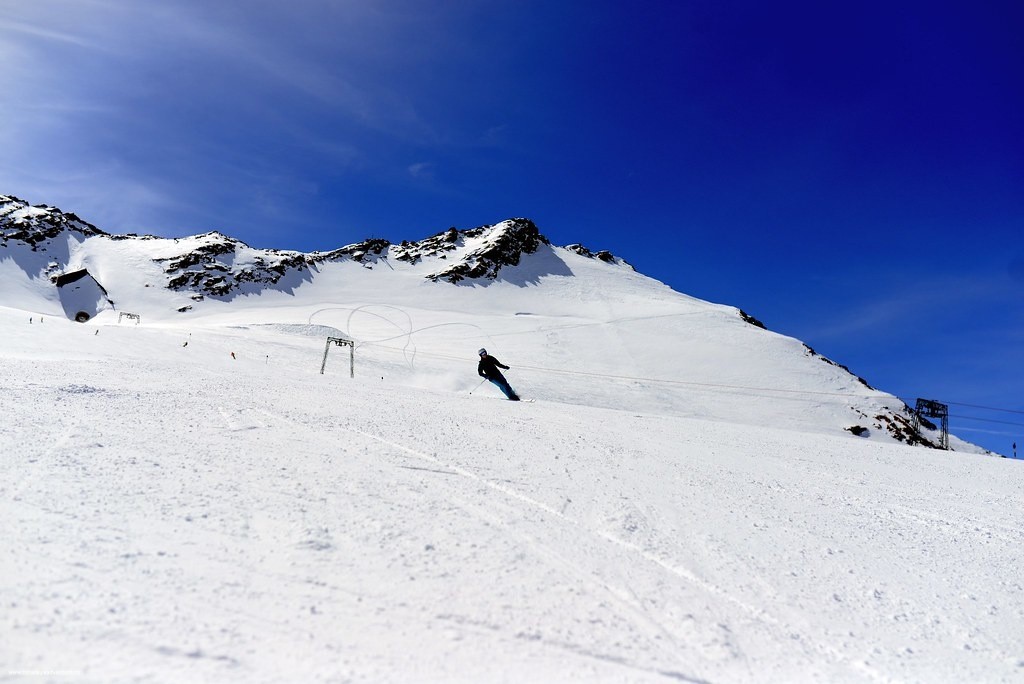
top-left (478, 348), bottom-right (486, 356)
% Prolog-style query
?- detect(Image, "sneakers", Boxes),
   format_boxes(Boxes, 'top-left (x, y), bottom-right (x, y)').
top-left (509, 395), bottom-right (520, 400)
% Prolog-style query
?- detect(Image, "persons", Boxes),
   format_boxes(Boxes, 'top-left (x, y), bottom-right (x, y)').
top-left (478, 348), bottom-right (520, 401)
top-left (231, 351), bottom-right (235, 359)
top-left (183, 342), bottom-right (187, 347)
